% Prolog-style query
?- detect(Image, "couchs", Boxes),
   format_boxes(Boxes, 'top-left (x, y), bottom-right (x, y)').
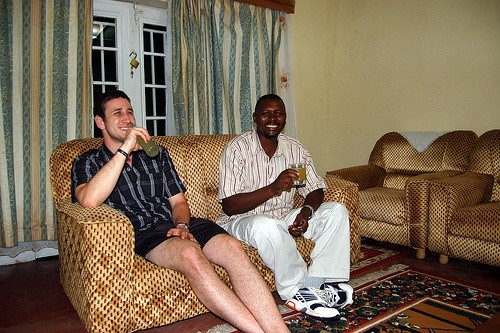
top-left (49, 128), bottom-right (500, 333)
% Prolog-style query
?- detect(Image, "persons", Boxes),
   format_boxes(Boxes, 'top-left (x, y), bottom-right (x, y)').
top-left (71, 89), bottom-right (291, 333)
top-left (218, 94), bottom-right (355, 318)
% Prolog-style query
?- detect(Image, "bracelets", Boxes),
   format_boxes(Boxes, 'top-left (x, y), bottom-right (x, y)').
top-left (176, 222), bottom-right (190, 229)
top-left (302, 205), bottom-right (314, 219)
top-left (117, 149), bottom-right (129, 157)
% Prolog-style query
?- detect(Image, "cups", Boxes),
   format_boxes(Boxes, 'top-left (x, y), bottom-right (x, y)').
top-left (288, 163), bottom-right (306, 186)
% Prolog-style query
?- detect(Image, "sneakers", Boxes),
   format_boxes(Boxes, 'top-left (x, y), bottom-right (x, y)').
top-left (319, 283), bottom-right (355, 310)
top-left (285, 287), bottom-right (340, 319)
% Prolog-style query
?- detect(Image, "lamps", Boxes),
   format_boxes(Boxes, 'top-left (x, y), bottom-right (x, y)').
top-left (92, 24), bottom-right (106, 39)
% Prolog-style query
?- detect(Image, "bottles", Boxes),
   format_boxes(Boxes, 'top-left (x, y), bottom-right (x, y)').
top-left (127, 121), bottom-right (159, 157)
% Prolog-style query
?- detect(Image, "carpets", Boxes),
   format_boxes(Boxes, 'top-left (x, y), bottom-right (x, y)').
top-left (194, 245), bottom-right (500, 333)
